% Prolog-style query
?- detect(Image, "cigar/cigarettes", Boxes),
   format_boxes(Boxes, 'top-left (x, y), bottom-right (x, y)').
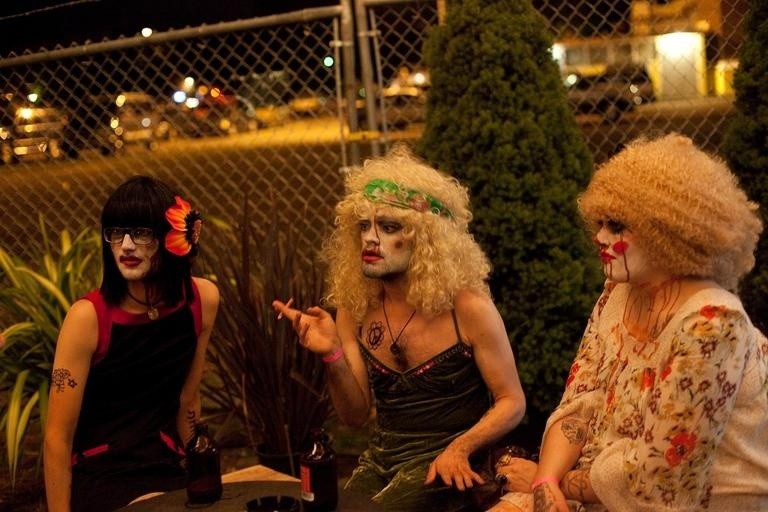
top-left (277, 294), bottom-right (296, 319)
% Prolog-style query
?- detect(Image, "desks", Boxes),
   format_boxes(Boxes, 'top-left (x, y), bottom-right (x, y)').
top-left (120, 465), bottom-right (309, 511)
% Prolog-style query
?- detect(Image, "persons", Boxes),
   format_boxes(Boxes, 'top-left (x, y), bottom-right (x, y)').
top-left (44, 177), bottom-right (220, 511)
top-left (0, 90), bottom-right (20, 165)
top-left (271, 141), bottom-right (527, 511)
top-left (489, 130), bottom-right (768, 512)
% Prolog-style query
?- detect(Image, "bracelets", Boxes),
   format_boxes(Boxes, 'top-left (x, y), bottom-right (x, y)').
top-left (322, 347), bottom-right (344, 363)
top-left (530, 476), bottom-right (560, 488)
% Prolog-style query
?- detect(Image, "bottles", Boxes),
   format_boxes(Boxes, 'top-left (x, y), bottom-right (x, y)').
top-left (185, 422), bottom-right (223, 503)
top-left (299, 426), bottom-right (337, 511)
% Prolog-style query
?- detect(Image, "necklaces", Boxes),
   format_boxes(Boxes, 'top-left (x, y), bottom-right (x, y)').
top-left (383, 294), bottom-right (417, 355)
top-left (127, 289), bottom-right (163, 321)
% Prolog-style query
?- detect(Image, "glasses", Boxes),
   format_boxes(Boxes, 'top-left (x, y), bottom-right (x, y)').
top-left (103, 226), bottom-right (154, 245)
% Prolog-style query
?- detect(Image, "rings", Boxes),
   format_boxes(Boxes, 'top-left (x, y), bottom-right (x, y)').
top-left (499, 455), bottom-right (511, 466)
top-left (494, 472), bottom-right (509, 484)
